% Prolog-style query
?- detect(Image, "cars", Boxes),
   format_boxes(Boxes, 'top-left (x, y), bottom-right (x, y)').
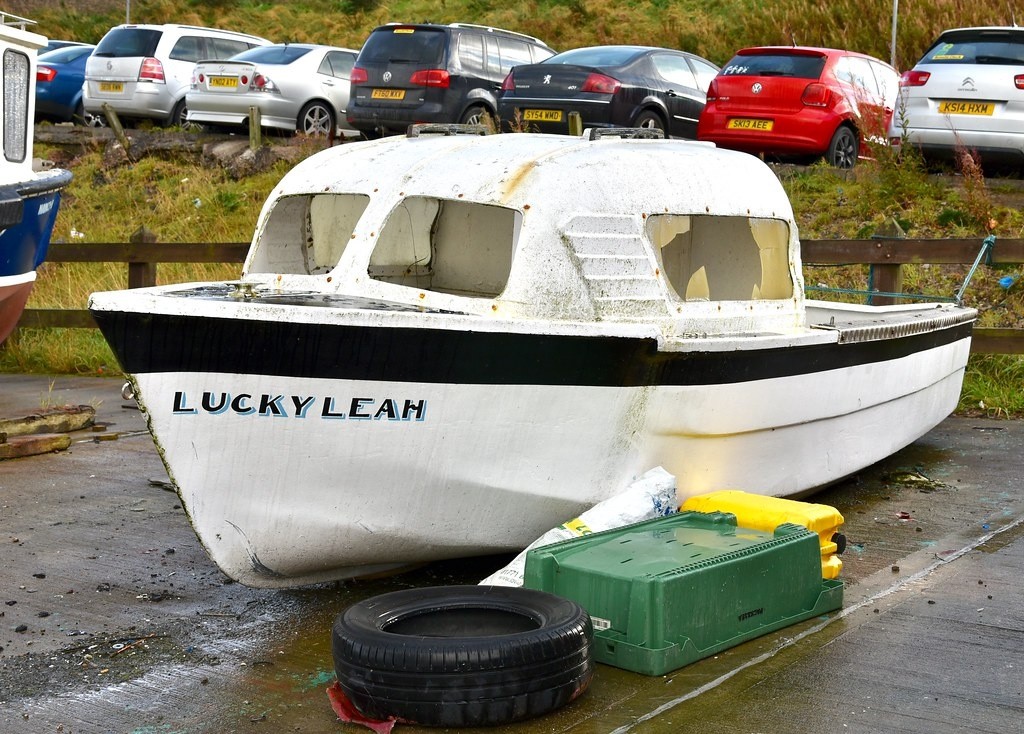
top-left (184, 42), bottom-right (364, 141)
top-left (888, 23), bottom-right (1024, 175)
top-left (35, 46), bottom-right (112, 129)
top-left (494, 45), bottom-right (726, 142)
top-left (696, 45), bottom-right (904, 175)
top-left (36, 39), bottom-right (92, 54)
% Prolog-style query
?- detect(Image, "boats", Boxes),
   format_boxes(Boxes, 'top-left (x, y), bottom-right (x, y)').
top-left (82, 129), bottom-right (980, 592)
top-left (0, 9), bottom-right (73, 349)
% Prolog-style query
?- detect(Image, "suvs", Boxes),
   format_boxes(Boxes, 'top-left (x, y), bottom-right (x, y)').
top-left (347, 20), bottom-right (562, 138)
top-left (81, 23), bottom-right (284, 133)
